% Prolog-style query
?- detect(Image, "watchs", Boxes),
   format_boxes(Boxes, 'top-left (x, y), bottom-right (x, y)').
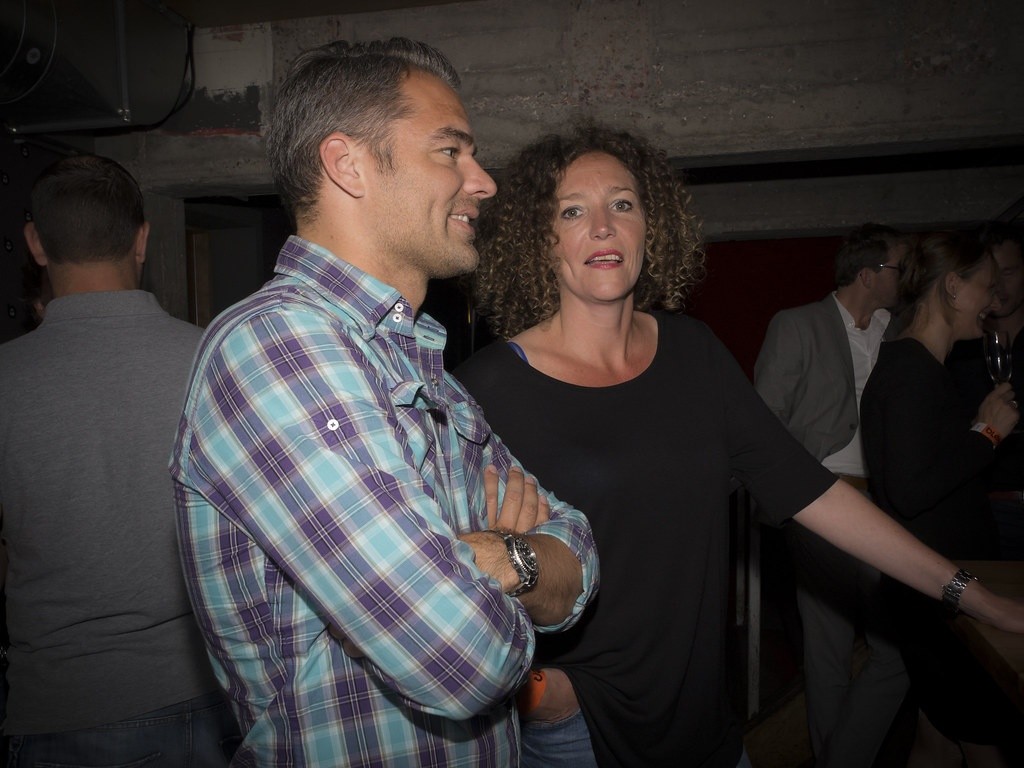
top-left (484, 526), bottom-right (539, 600)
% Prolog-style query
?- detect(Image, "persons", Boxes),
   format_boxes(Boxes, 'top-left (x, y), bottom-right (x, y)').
top-left (860, 233), bottom-right (1021, 768)
top-left (168, 35), bottom-right (601, 766)
top-left (446, 120), bottom-right (1023, 763)
top-left (947, 223), bottom-right (1024, 455)
top-left (0, 148), bottom-right (255, 768)
top-left (744, 218), bottom-right (913, 768)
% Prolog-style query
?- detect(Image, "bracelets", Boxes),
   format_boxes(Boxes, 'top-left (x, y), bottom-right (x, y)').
top-left (515, 666), bottom-right (548, 718)
top-left (939, 568), bottom-right (980, 611)
top-left (972, 422), bottom-right (1004, 447)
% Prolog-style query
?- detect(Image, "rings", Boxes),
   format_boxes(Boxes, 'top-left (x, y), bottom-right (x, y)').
top-left (1010, 400), bottom-right (1018, 409)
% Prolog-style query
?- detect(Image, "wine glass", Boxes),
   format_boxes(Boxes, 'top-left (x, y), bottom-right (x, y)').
top-left (983, 329), bottom-right (1012, 386)
